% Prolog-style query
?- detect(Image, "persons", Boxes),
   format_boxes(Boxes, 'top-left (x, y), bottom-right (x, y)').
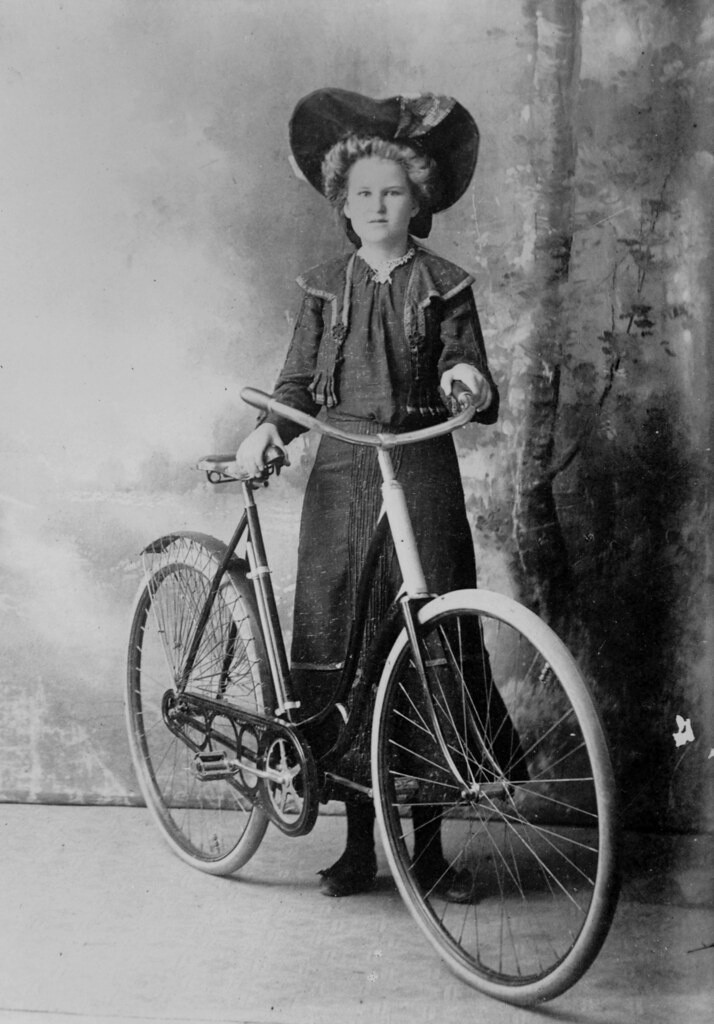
top-left (235, 87), bottom-right (530, 903)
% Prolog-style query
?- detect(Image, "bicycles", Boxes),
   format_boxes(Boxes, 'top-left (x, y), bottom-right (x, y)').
top-left (126, 377), bottom-right (628, 1009)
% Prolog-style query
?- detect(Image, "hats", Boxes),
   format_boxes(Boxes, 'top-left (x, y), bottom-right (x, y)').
top-left (288, 88), bottom-right (481, 239)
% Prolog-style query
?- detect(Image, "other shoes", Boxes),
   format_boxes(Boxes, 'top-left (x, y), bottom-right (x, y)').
top-left (410, 856), bottom-right (477, 902)
top-left (318, 852), bottom-right (378, 898)
